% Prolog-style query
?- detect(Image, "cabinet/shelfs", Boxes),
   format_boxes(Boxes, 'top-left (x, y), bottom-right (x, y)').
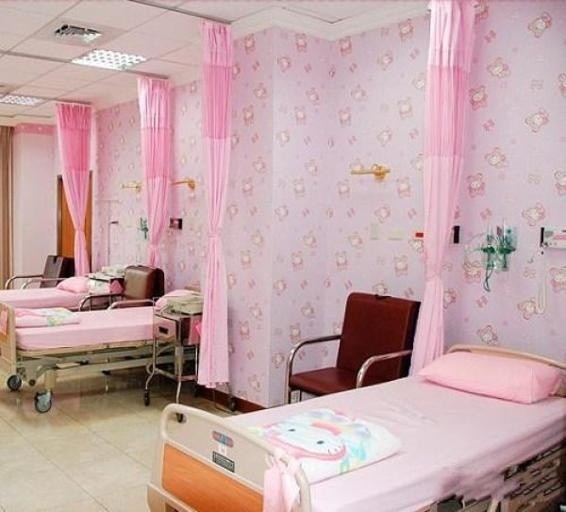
top-left (143, 314), bottom-right (238, 423)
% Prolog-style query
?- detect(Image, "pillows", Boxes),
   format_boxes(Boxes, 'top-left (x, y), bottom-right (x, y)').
top-left (156, 287), bottom-right (207, 315)
top-left (418, 351), bottom-right (564, 406)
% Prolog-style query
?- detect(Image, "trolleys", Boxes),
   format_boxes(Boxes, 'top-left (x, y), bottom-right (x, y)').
top-left (143, 295), bottom-right (237, 422)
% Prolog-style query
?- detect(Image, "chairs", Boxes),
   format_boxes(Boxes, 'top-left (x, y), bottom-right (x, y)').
top-left (283, 292), bottom-right (422, 406)
top-left (4, 253), bottom-right (75, 289)
top-left (79, 264), bottom-right (164, 311)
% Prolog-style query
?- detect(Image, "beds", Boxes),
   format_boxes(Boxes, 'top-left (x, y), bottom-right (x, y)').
top-left (146, 343), bottom-right (566, 512)
top-left (0, 274), bottom-right (152, 412)
